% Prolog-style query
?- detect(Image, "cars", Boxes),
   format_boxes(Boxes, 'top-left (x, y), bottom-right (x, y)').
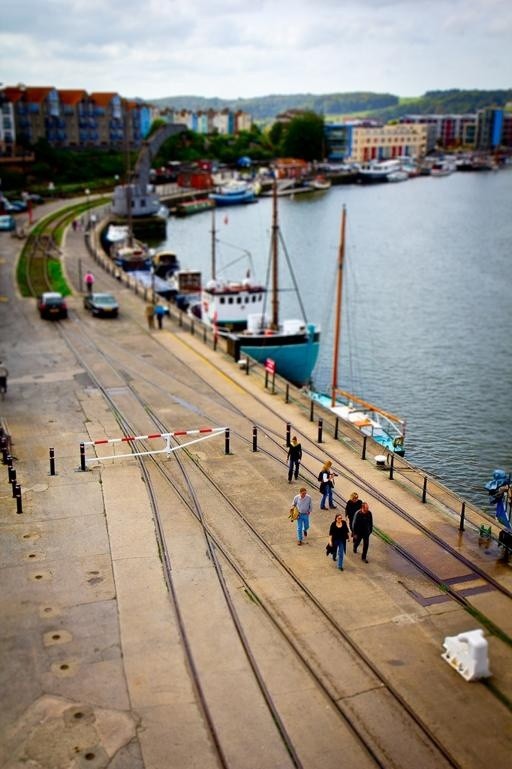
top-left (0, 215), bottom-right (16, 230)
top-left (84, 293), bottom-right (119, 318)
top-left (37, 291), bottom-right (69, 322)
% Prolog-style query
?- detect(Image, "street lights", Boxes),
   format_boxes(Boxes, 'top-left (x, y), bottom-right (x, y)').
top-left (84, 188), bottom-right (93, 222)
top-left (88, 214), bottom-right (101, 262)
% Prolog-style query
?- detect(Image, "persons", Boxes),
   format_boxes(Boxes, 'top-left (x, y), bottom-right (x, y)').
top-left (290, 487), bottom-right (313, 545)
top-left (344, 492), bottom-right (373, 564)
top-left (84, 271), bottom-right (94, 293)
top-left (318, 460), bottom-right (337, 510)
top-left (145, 305), bottom-right (164, 330)
top-left (81, 215), bottom-right (84, 225)
top-left (285, 437), bottom-right (302, 483)
top-left (329, 514), bottom-right (350, 572)
top-left (72, 219), bottom-right (77, 231)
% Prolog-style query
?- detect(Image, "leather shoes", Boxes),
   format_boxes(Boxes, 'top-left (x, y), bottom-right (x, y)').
top-left (297, 541), bottom-right (301, 545)
top-left (304, 531), bottom-right (307, 536)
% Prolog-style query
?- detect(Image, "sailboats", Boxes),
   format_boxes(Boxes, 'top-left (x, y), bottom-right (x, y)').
top-left (308, 203), bottom-right (404, 458)
top-left (187, 177), bottom-right (322, 385)
top-left (107, 103), bottom-right (168, 237)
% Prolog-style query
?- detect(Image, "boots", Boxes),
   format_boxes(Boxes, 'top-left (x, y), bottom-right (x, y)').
top-left (330, 505), bottom-right (337, 509)
top-left (321, 507), bottom-right (328, 510)
top-left (338, 567), bottom-right (343, 571)
top-left (362, 559), bottom-right (368, 563)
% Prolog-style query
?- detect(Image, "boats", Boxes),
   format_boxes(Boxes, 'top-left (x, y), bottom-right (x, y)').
top-left (206, 159), bottom-right (457, 202)
top-left (108, 184), bottom-right (180, 276)
